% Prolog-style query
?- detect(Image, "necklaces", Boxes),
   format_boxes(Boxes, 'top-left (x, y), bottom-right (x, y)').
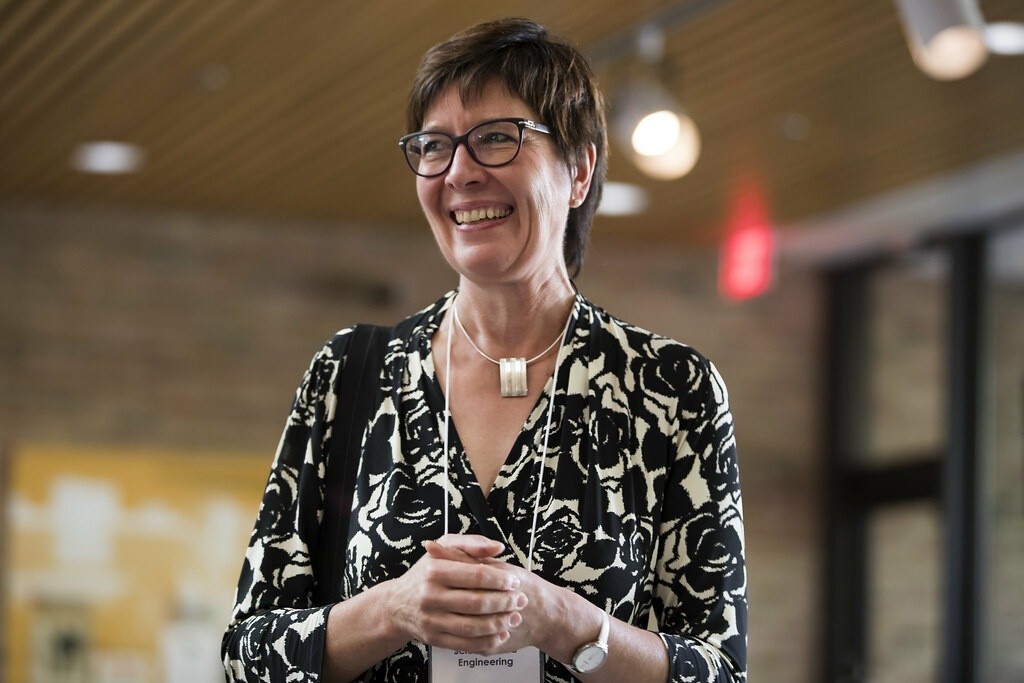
top-left (441, 292), bottom-right (565, 397)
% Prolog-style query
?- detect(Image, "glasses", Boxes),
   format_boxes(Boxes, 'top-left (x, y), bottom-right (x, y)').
top-left (398, 117), bottom-right (553, 178)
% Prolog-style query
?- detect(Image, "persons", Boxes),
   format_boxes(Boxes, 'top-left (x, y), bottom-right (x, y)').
top-left (222, 16), bottom-right (747, 683)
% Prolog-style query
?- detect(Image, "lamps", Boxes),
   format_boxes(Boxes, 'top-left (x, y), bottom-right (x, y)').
top-left (893, 0), bottom-right (990, 84)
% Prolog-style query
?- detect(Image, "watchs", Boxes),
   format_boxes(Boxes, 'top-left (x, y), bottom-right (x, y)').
top-left (561, 609), bottom-right (609, 680)
